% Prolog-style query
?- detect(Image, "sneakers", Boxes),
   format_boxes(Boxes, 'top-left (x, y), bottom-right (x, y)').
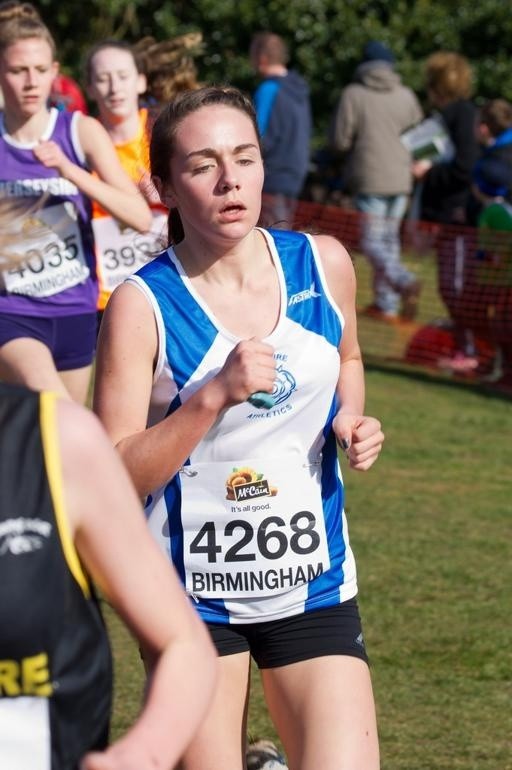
top-left (242, 732), bottom-right (288, 770)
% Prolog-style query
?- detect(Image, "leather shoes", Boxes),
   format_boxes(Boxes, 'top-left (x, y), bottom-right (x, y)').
top-left (361, 304), bottom-right (401, 325)
top-left (398, 282), bottom-right (420, 327)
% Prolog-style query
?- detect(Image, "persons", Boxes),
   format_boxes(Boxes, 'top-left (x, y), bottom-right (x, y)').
top-left (92, 87), bottom-right (388, 770)
top-left (467, 160), bottom-right (512, 386)
top-left (0, 5), bottom-right (152, 413)
top-left (0, 379), bottom-right (220, 770)
top-left (79, 41), bottom-right (171, 327)
top-left (472, 97), bottom-right (511, 233)
top-left (337, 37), bottom-right (426, 322)
top-left (254, 32), bottom-right (311, 231)
top-left (410, 52), bottom-right (485, 373)
